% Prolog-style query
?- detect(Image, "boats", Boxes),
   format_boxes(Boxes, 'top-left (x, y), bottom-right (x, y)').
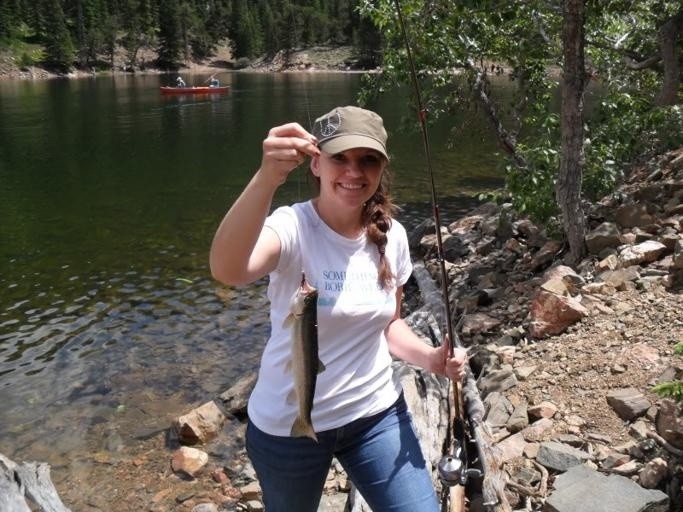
top-left (160, 86), bottom-right (230, 94)
top-left (161, 94), bottom-right (230, 99)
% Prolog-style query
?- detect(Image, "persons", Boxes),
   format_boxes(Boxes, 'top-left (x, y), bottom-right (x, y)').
top-left (90, 66), bottom-right (95, 79)
top-left (176, 76), bottom-right (184, 87)
top-left (209, 76), bottom-right (218, 88)
top-left (209, 105), bottom-right (465, 512)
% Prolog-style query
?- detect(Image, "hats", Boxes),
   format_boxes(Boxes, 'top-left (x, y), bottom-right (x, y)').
top-left (311, 106), bottom-right (389, 163)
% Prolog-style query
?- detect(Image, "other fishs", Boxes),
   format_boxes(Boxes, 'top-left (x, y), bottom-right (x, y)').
top-left (279, 277), bottom-right (326, 444)
